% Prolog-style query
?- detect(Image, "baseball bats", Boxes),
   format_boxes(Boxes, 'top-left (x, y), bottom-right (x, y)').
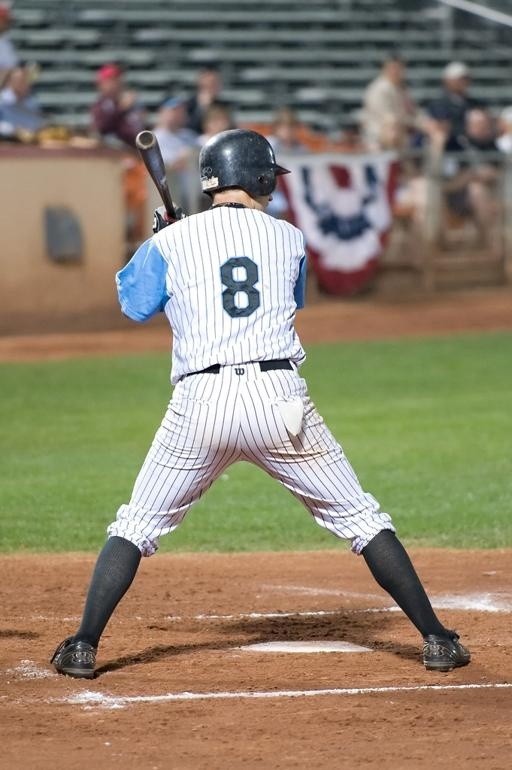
top-left (136, 130), bottom-right (176, 225)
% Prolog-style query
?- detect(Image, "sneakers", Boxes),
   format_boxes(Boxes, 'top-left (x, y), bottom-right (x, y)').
top-left (50, 637), bottom-right (97, 678)
top-left (424, 630), bottom-right (471, 670)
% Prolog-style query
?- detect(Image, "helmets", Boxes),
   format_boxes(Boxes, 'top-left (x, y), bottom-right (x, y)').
top-left (199, 129), bottom-right (291, 196)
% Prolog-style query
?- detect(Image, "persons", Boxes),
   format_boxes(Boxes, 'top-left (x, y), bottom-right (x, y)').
top-left (49, 126), bottom-right (470, 678)
top-left (1, 1), bottom-right (48, 141)
top-left (74, 58), bottom-right (511, 278)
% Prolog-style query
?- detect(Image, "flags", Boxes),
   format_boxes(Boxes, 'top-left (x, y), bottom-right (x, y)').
top-left (272, 152), bottom-right (404, 299)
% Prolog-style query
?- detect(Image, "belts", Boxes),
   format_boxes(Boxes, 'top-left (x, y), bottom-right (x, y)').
top-left (186, 357), bottom-right (298, 378)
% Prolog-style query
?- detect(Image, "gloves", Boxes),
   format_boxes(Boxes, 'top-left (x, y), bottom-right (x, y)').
top-left (153, 201), bottom-right (186, 233)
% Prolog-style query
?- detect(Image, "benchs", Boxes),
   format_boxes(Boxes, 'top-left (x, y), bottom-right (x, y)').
top-left (7, 1), bottom-right (512, 162)
